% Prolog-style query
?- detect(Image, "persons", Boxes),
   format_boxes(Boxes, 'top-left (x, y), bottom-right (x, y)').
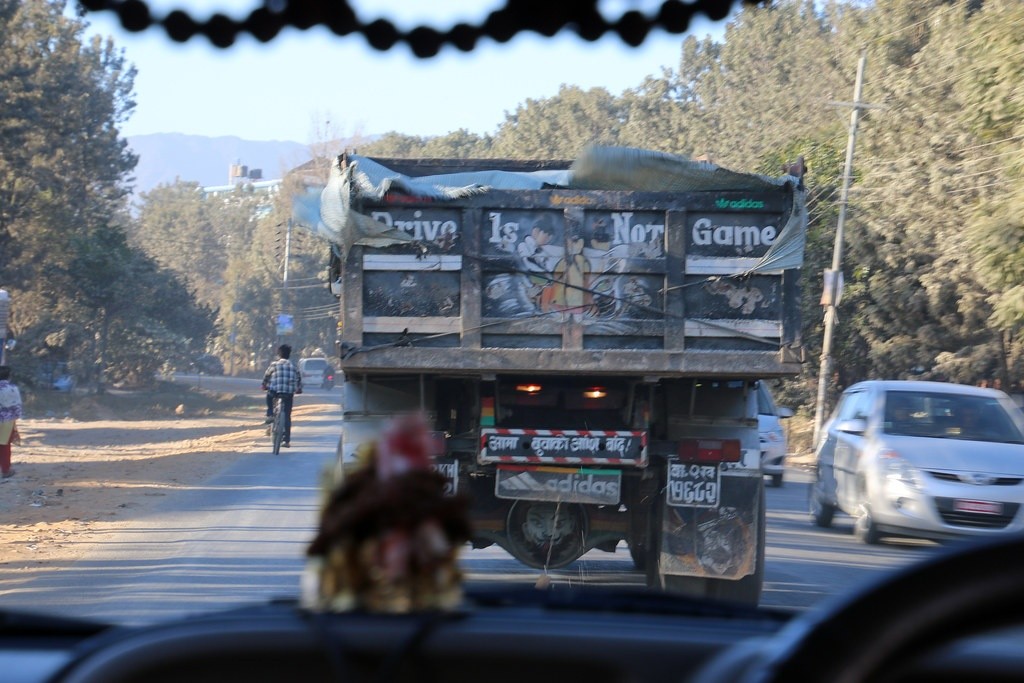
top-left (953, 401), bottom-right (987, 431)
top-left (263, 344), bottom-right (302, 448)
top-left (320, 364), bottom-right (335, 390)
top-left (891, 398), bottom-right (921, 436)
top-left (0, 366), bottom-right (22, 479)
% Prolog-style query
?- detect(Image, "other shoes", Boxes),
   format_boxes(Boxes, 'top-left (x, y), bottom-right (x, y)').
top-left (0, 469), bottom-right (16, 478)
top-left (281, 442), bottom-right (290, 448)
top-left (266, 417), bottom-right (275, 424)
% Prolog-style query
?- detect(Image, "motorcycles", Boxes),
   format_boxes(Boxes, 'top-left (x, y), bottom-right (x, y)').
top-left (320, 376), bottom-right (335, 391)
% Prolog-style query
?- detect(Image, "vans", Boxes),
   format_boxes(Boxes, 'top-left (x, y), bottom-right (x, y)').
top-left (298, 358), bottom-right (327, 386)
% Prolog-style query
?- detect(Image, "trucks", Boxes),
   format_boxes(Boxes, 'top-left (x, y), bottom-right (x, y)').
top-left (330, 150), bottom-right (809, 614)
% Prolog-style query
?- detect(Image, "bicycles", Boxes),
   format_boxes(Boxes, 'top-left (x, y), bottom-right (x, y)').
top-left (264, 387), bottom-right (302, 455)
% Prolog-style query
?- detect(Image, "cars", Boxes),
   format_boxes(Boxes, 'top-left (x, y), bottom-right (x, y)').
top-left (698, 381), bottom-right (792, 488)
top-left (810, 381), bottom-right (1024, 543)
